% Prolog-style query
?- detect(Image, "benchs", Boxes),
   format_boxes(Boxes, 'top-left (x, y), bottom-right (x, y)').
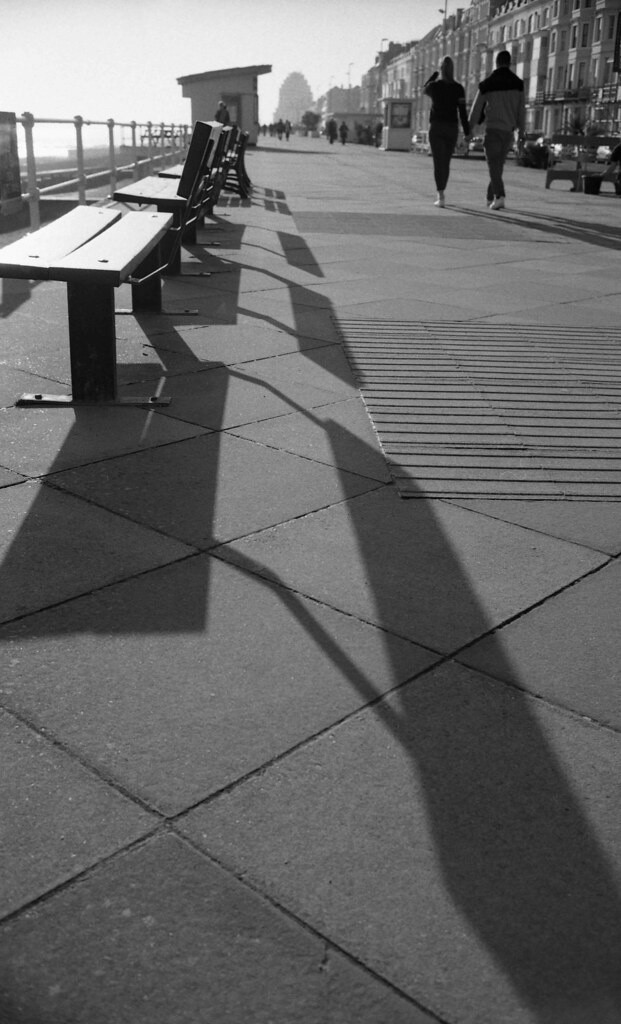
top-left (0, 120), bottom-right (252, 408)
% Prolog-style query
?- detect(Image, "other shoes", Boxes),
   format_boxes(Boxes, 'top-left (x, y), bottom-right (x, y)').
top-left (489, 195), bottom-right (504, 209)
top-left (432, 189), bottom-right (445, 206)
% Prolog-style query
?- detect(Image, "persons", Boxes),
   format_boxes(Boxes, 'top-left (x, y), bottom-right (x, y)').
top-left (422, 55), bottom-right (473, 209)
top-left (261, 119), bottom-right (293, 141)
top-left (463, 51), bottom-right (527, 199)
top-left (356, 123), bottom-right (383, 147)
top-left (339, 121), bottom-right (349, 145)
top-left (215, 101), bottom-right (230, 127)
top-left (325, 117), bottom-right (338, 144)
